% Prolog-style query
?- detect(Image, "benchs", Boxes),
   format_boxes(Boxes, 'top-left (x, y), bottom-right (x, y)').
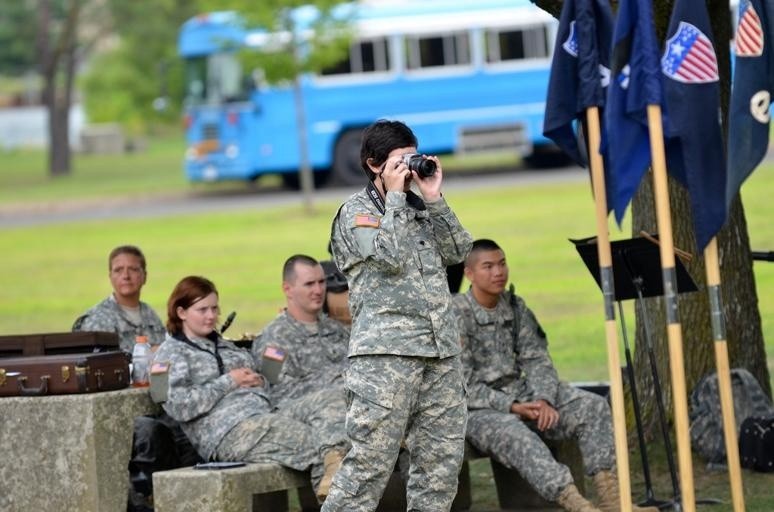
top-left (151, 421), bottom-right (586, 512)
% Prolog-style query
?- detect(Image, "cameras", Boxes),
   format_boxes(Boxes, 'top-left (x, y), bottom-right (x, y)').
top-left (394, 153), bottom-right (437, 179)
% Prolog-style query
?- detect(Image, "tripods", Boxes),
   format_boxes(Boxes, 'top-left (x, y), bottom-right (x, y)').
top-left (633, 278), bottom-right (723, 511)
top-left (617, 301), bottom-right (668, 508)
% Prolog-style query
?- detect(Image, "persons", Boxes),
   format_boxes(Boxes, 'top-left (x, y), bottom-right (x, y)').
top-left (251, 255), bottom-right (411, 489)
top-left (452, 239), bottom-right (659, 512)
top-left (79, 246), bottom-right (204, 487)
top-left (320, 119), bottom-right (474, 512)
top-left (147, 276), bottom-right (351, 506)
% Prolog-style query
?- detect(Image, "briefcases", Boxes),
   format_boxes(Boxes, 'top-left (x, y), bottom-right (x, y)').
top-left (0, 330), bottom-right (132, 397)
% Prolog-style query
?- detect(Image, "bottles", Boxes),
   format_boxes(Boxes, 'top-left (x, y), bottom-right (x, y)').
top-left (132, 336), bottom-right (151, 386)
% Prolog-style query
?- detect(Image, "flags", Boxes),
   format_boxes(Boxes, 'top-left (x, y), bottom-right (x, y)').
top-left (530, 0), bottom-right (774, 257)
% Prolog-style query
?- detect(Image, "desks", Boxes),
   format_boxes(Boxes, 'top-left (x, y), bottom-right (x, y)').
top-left (0, 384), bottom-right (166, 512)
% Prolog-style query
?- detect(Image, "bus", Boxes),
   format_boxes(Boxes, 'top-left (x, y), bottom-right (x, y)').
top-left (181, 1), bottom-right (593, 188)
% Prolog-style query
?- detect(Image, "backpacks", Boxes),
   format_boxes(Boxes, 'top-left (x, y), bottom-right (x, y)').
top-left (686, 367), bottom-right (774, 464)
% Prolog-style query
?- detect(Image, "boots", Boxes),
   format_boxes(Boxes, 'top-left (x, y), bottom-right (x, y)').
top-left (584, 470), bottom-right (658, 512)
top-left (556, 484), bottom-right (603, 512)
top-left (315, 452), bottom-right (344, 501)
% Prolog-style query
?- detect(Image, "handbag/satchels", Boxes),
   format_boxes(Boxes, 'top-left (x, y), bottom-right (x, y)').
top-left (738, 416), bottom-right (774, 475)
top-left (126, 412), bottom-right (201, 496)
